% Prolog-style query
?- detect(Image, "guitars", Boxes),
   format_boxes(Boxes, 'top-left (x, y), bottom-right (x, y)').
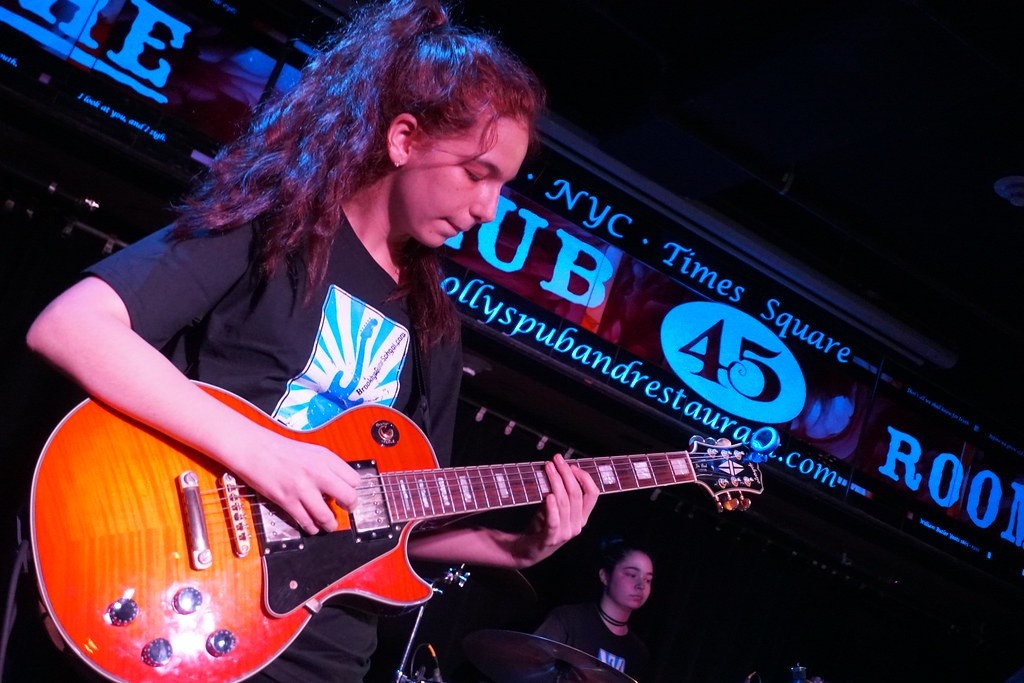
top-left (30, 381), bottom-right (764, 682)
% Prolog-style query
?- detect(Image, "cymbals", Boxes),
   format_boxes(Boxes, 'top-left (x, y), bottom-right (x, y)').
top-left (472, 565), bottom-right (541, 606)
top-left (462, 629), bottom-right (639, 683)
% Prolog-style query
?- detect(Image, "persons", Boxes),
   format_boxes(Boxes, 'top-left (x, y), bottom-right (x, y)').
top-left (26, 0), bottom-right (600, 683)
top-left (531, 541), bottom-right (653, 683)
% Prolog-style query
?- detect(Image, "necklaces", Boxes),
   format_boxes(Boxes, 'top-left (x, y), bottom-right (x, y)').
top-left (599, 607), bottom-right (627, 627)
